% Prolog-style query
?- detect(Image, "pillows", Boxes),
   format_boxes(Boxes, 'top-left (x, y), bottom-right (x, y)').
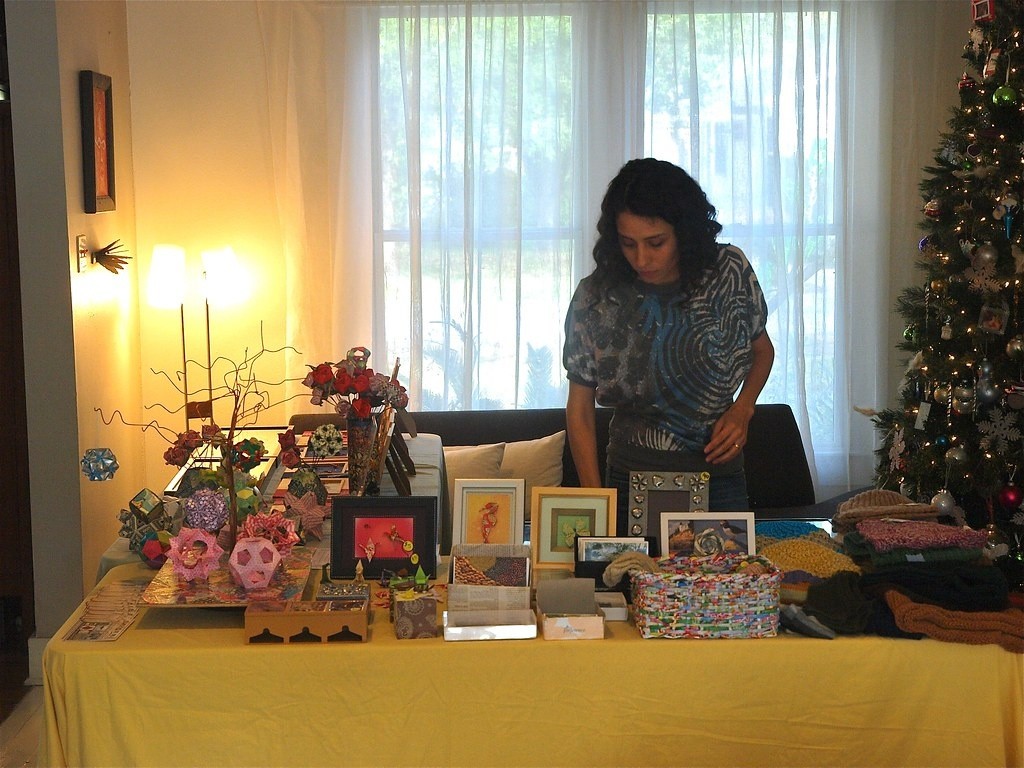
top-left (501, 429), bottom-right (566, 522)
top-left (442, 442), bottom-right (507, 521)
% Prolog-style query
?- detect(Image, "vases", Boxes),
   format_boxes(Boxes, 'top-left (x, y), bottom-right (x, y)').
top-left (374, 423), bottom-right (395, 495)
top-left (351, 422), bottom-right (379, 498)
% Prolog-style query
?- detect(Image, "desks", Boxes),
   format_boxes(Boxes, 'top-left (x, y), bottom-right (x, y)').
top-left (44, 564), bottom-right (1024, 768)
top-left (93, 434), bottom-right (450, 584)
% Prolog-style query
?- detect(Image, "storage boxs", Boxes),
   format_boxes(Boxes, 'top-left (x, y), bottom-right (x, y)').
top-left (444, 544), bottom-right (628, 644)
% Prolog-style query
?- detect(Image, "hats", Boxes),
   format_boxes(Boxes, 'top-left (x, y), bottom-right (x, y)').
top-left (837, 489), bottom-right (940, 527)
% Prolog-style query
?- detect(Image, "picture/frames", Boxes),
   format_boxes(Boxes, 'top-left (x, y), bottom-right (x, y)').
top-left (660, 511), bottom-right (756, 558)
top-left (627, 471), bottom-right (710, 560)
top-left (576, 538), bottom-right (656, 595)
top-left (451, 479), bottom-right (524, 545)
top-left (531, 487), bottom-right (618, 588)
top-left (330, 495), bottom-right (438, 580)
top-left (162, 424), bottom-right (351, 516)
top-left (79, 69), bottom-right (116, 214)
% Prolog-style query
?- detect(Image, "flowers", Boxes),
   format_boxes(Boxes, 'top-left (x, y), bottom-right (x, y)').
top-left (302, 347), bottom-right (410, 425)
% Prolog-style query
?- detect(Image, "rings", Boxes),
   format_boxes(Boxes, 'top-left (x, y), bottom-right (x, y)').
top-left (733, 444), bottom-right (740, 449)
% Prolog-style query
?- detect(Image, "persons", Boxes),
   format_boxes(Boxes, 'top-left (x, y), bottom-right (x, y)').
top-left (562, 157), bottom-right (775, 557)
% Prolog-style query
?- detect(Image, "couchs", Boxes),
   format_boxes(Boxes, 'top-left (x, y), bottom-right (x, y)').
top-left (288, 404), bottom-right (815, 523)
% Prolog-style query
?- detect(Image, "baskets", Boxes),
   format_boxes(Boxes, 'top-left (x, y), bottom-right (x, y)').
top-left (629, 554), bottom-right (782, 639)
